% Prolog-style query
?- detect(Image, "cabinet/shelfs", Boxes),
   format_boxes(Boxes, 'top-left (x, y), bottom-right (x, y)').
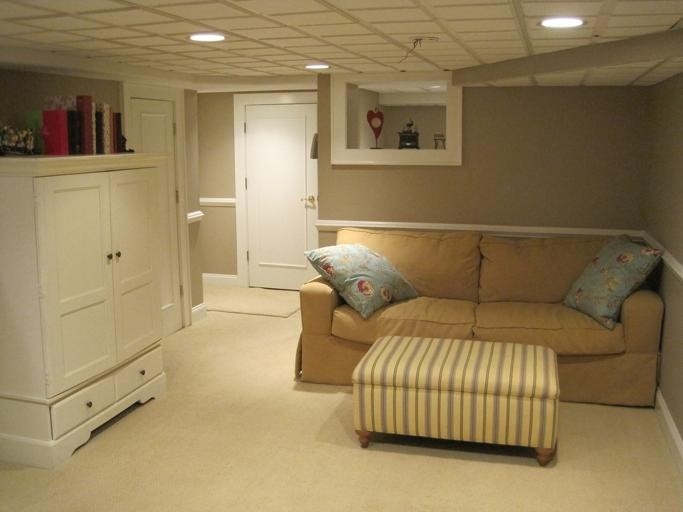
top-left (0, 153), bottom-right (166, 466)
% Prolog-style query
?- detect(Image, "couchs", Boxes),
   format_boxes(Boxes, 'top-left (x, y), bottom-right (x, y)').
top-left (301, 227), bottom-right (665, 407)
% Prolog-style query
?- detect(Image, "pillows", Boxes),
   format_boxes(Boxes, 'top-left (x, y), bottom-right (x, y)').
top-left (562, 236), bottom-right (664, 331)
top-left (305, 244), bottom-right (418, 320)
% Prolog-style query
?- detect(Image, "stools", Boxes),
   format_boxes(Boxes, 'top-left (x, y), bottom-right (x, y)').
top-left (351, 335), bottom-right (560, 466)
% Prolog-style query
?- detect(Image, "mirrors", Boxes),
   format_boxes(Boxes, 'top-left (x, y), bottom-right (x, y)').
top-left (331, 72), bottom-right (462, 167)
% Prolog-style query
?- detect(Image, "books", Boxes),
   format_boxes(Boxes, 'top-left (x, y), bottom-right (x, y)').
top-left (27, 95), bottom-right (122, 155)
